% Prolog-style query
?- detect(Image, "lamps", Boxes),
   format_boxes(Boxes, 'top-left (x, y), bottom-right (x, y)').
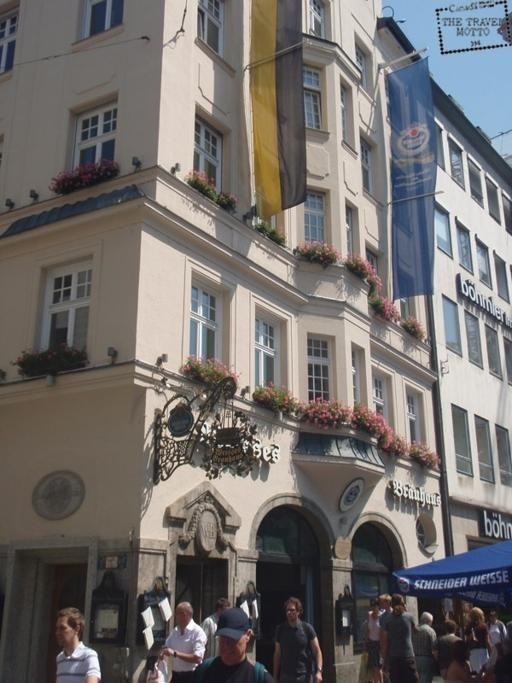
top-left (241, 386), bottom-right (249, 396)
top-left (157, 353), bottom-right (168, 365)
top-left (30, 189), bottom-right (38, 200)
top-left (132, 157), bottom-right (141, 167)
top-left (108, 346), bottom-right (117, 359)
top-left (171, 162), bottom-right (180, 173)
top-left (5, 198), bottom-right (14, 208)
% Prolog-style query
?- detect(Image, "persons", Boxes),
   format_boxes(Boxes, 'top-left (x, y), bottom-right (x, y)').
top-left (160, 597), bottom-right (324, 683)
top-left (55, 607), bottom-right (101, 683)
top-left (367, 592), bottom-right (512, 683)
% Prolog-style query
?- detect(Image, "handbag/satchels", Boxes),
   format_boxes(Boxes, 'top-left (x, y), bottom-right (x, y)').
top-left (147, 660), bottom-right (169, 683)
top-left (358, 653), bottom-right (383, 683)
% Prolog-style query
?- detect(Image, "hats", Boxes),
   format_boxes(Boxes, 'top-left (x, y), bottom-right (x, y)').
top-left (214, 607), bottom-right (250, 640)
